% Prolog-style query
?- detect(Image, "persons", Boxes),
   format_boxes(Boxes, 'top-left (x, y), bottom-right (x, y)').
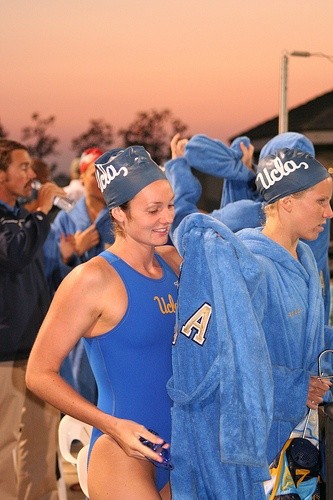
top-left (58, 147), bottom-right (112, 258)
top-left (211, 147), bottom-right (333, 500)
top-left (169, 130), bottom-right (315, 213)
top-left (25, 145), bottom-right (185, 499)
top-left (0, 138), bottom-right (63, 499)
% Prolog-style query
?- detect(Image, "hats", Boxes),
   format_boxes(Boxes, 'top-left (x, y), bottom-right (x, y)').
top-left (78, 145), bottom-right (103, 173)
top-left (255, 149), bottom-right (329, 204)
top-left (93, 146), bottom-right (167, 212)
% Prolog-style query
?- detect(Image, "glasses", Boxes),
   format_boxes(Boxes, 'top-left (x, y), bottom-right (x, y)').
top-left (139, 429), bottom-right (174, 470)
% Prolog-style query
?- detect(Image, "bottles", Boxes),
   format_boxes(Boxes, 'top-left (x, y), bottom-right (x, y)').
top-left (29, 179), bottom-right (78, 212)
